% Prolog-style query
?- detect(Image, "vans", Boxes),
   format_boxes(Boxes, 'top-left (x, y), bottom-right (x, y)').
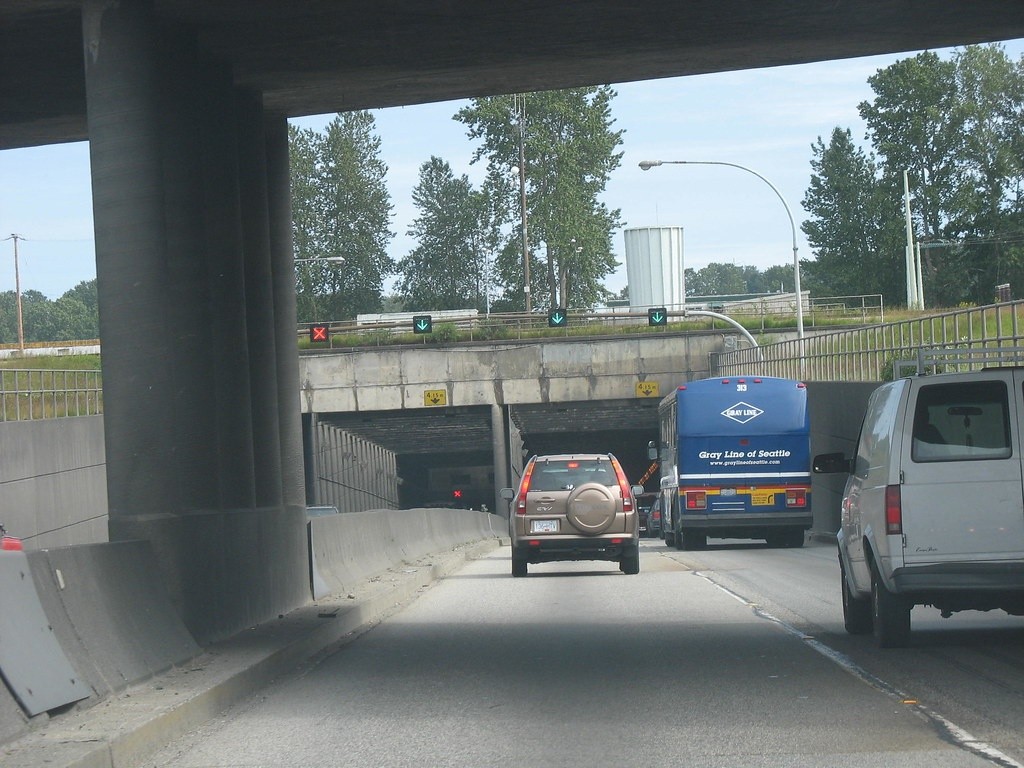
top-left (810, 363), bottom-right (1024, 647)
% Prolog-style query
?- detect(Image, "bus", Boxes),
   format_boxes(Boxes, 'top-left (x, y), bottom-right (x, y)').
top-left (648, 375), bottom-right (813, 551)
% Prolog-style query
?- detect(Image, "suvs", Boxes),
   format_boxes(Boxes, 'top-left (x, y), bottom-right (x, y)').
top-left (499, 454), bottom-right (646, 578)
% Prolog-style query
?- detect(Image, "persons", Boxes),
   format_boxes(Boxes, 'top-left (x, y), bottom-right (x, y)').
top-left (911, 397), bottom-right (952, 460)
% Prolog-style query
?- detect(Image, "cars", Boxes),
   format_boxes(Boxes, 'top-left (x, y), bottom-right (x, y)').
top-left (646, 498), bottom-right (661, 539)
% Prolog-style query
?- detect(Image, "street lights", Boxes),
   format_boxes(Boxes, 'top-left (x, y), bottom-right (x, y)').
top-left (639, 160), bottom-right (807, 384)
